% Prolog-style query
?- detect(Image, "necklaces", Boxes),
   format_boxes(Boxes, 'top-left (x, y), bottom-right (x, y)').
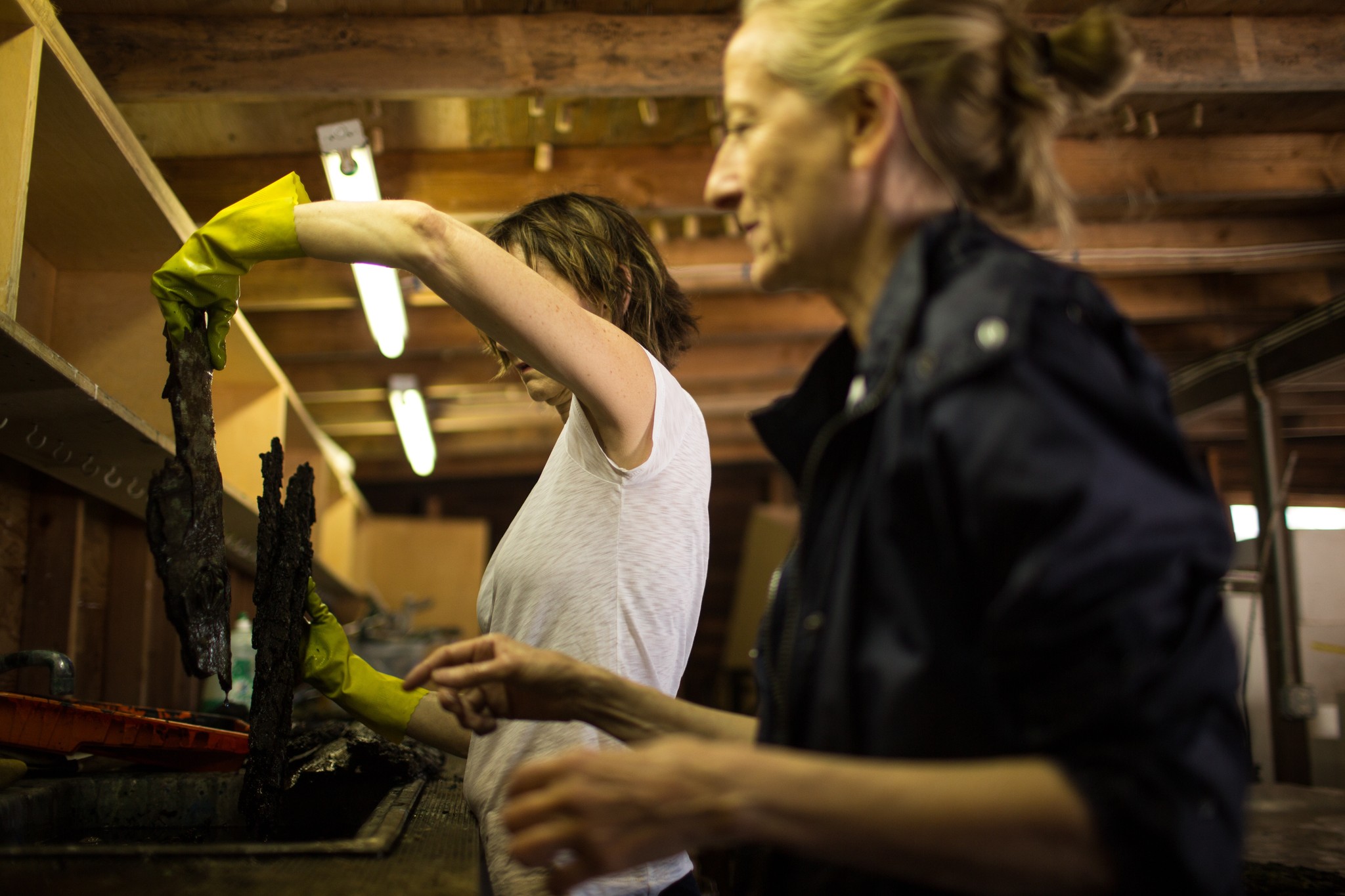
top-left (151, 171), bottom-right (697, 896)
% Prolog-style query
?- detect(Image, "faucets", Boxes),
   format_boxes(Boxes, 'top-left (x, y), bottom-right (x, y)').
top-left (6, 647), bottom-right (76, 696)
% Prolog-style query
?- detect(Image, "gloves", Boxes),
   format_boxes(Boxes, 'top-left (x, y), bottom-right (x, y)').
top-left (147, 171), bottom-right (308, 370)
top-left (253, 573), bottom-right (430, 734)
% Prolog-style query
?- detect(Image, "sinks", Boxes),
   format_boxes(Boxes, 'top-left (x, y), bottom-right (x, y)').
top-left (0, 772), bottom-right (429, 856)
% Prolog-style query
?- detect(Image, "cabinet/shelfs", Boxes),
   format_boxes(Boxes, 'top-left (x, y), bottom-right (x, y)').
top-left (0, 0), bottom-right (371, 595)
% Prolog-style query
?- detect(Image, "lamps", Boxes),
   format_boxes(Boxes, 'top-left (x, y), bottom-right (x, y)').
top-left (311, 117), bottom-right (417, 362)
top-left (382, 371), bottom-right (442, 484)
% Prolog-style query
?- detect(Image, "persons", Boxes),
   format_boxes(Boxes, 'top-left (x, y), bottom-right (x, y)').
top-left (402, 0), bottom-right (1262, 896)
